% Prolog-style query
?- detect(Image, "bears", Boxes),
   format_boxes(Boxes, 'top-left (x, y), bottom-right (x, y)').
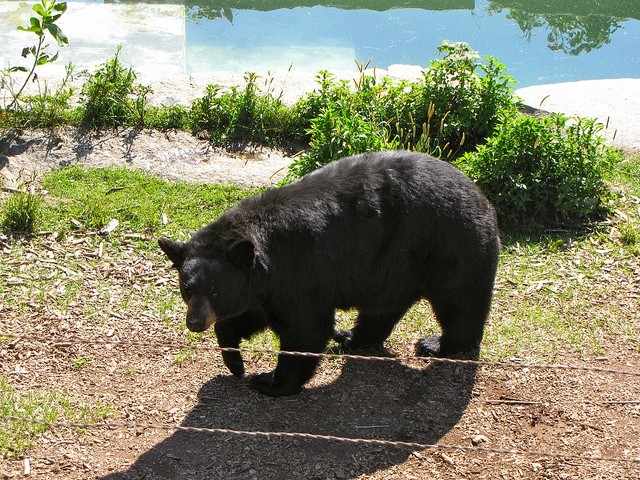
top-left (158, 149), bottom-right (502, 398)
